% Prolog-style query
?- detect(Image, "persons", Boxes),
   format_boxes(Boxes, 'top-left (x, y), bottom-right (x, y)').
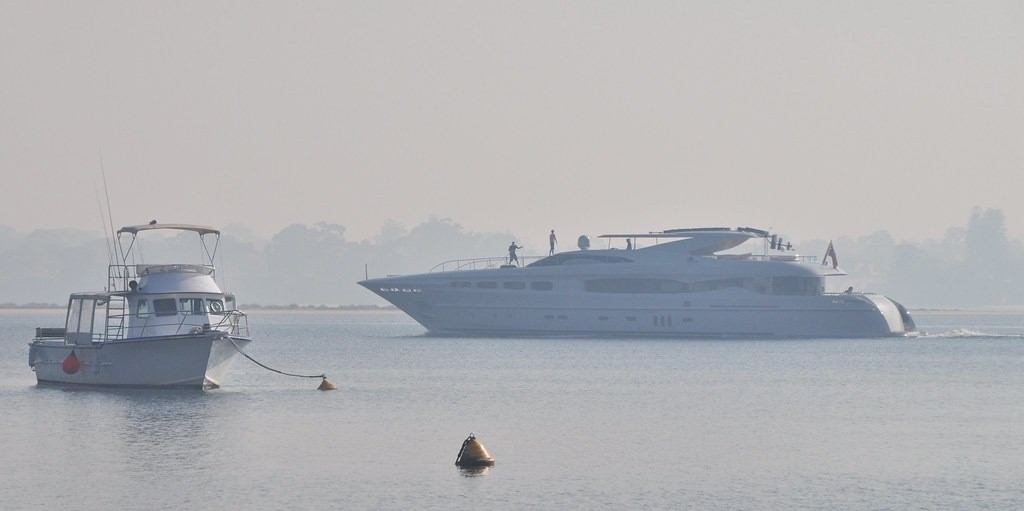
top-left (508, 241), bottom-right (523, 267)
top-left (828, 241), bottom-right (839, 269)
top-left (626, 239), bottom-right (632, 250)
top-left (549, 230), bottom-right (558, 255)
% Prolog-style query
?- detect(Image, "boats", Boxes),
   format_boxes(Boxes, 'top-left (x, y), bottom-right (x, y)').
top-left (357, 228), bottom-right (921, 339)
top-left (26, 151), bottom-right (253, 390)
